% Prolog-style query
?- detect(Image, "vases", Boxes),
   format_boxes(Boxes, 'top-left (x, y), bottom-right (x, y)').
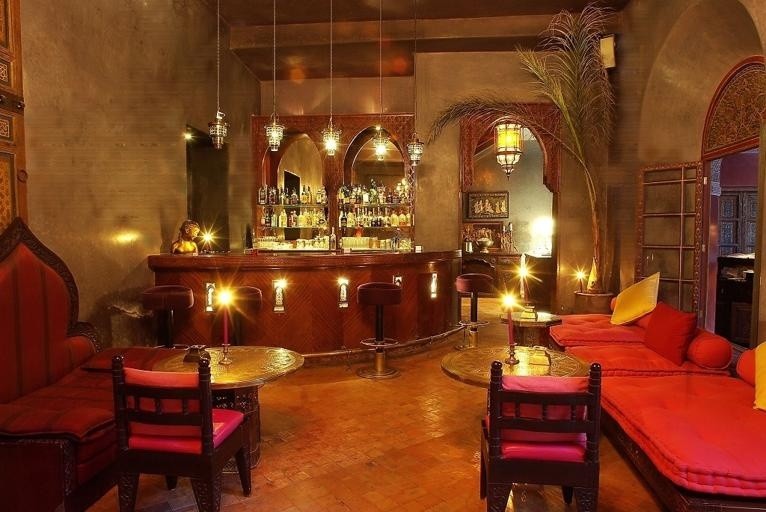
top-left (573, 290), bottom-right (614, 314)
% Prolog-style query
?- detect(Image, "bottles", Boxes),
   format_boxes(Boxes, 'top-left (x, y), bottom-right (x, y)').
top-left (258, 182), bottom-right (414, 251)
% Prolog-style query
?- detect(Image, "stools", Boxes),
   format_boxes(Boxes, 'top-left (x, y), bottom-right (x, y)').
top-left (141, 285), bottom-right (194, 350)
top-left (356, 281), bottom-right (402, 380)
top-left (453, 273), bottom-right (493, 352)
top-left (212, 287), bottom-right (262, 347)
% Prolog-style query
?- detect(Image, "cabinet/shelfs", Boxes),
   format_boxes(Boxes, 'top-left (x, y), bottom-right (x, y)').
top-left (336, 203), bottom-right (416, 253)
top-left (252, 202), bottom-right (329, 253)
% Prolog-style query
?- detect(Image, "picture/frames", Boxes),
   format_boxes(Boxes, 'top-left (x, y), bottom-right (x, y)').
top-left (462, 220), bottom-right (505, 251)
top-left (467, 190), bottom-right (509, 219)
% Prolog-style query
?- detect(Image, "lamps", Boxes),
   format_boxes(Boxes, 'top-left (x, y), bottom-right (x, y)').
top-left (209, 0), bottom-right (424, 166)
top-left (493, 118), bottom-right (520, 184)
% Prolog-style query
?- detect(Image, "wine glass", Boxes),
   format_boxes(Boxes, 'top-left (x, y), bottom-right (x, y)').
top-left (476, 240), bottom-right (494, 254)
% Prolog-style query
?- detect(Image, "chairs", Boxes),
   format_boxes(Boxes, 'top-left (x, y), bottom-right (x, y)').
top-left (0, 216), bottom-right (188, 511)
top-left (111, 354), bottom-right (251, 511)
top-left (480, 362), bottom-right (601, 512)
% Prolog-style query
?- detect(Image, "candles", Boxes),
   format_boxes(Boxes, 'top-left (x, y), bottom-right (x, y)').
top-left (523, 276), bottom-right (529, 306)
top-left (580, 278), bottom-right (582, 293)
top-left (503, 225), bottom-right (505, 232)
top-left (508, 307), bottom-right (515, 345)
top-left (224, 305), bottom-right (228, 344)
top-left (509, 222), bottom-right (513, 230)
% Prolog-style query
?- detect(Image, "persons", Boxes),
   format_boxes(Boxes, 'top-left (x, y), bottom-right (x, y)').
top-left (171, 219), bottom-right (200, 253)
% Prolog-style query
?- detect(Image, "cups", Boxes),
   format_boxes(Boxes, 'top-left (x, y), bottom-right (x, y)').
top-left (465, 241), bottom-right (473, 252)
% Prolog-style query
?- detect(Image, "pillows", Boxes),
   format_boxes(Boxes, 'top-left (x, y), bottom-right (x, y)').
top-left (610, 271), bottom-right (660, 327)
top-left (483, 372), bottom-right (591, 444)
top-left (123, 365), bottom-right (203, 439)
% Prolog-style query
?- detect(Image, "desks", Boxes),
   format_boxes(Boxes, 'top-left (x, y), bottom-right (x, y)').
top-left (151, 344), bottom-right (305, 476)
top-left (499, 311), bottom-right (562, 346)
top-left (523, 253), bottom-right (554, 312)
top-left (441, 344), bottom-right (589, 417)
top-left (463, 252), bottom-right (522, 301)
